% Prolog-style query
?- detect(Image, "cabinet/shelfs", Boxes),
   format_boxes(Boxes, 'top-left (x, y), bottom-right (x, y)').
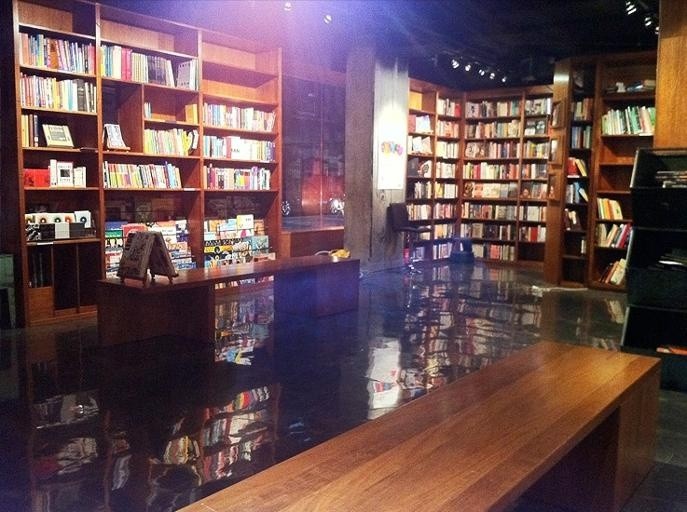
top-left (589, 46), bottom-right (658, 292)
top-left (16, 285), bottom-right (276, 512)
top-left (0, 1), bottom-right (282, 330)
top-left (464, 85), bottom-right (553, 273)
top-left (621, 147), bottom-right (687, 390)
top-left (402, 267), bottom-right (560, 404)
top-left (542, 57), bottom-right (597, 288)
top-left (404, 76), bottom-right (463, 265)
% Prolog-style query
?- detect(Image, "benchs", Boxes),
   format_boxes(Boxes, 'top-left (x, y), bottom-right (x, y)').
top-left (168, 339), bottom-right (661, 511)
top-left (94, 254), bottom-right (361, 350)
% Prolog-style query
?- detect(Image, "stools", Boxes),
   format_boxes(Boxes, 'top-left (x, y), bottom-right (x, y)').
top-left (0, 253), bottom-right (17, 329)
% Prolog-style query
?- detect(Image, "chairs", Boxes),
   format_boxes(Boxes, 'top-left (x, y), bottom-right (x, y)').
top-left (389, 203), bottom-right (431, 276)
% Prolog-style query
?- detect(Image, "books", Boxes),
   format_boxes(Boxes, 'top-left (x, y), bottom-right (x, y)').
top-left (463, 181), bottom-right (518, 198)
top-left (31, 252), bottom-right (45, 287)
top-left (432, 298), bottom-right (452, 312)
top-left (102, 123), bottom-right (131, 152)
top-left (146, 231), bottom-right (177, 279)
top-left (488, 282), bottom-right (510, 303)
top-left (407, 224), bottom-right (431, 241)
top-left (519, 206), bottom-right (547, 222)
top-left (460, 222), bottom-right (516, 241)
top-left (524, 96), bottom-right (551, 115)
top-left (458, 303), bottom-right (488, 318)
top-left (521, 182), bottom-right (547, 200)
top-left (202, 101), bottom-right (276, 132)
top-left (100, 43), bottom-right (199, 91)
top-left (201, 418), bottom-right (228, 448)
top-left (465, 99), bottom-right (521, 118)
top-left (19, 71), bottom-right (96, 114)
top-left (209, 386), bottom-right (272, 418)
top-left (407, 135), bottom-right (433, 156)
top-left (603, 298), bottom-right (625, 324)
top-left (436, 140), bottom-right (459, 159)
top-left (229, 408), bottom-right (269, 437)
top-left (161, 435), bottom-right (201, 466)
top-left (102, 160), bottom-right (182, 190)
top-left (596, 198), bottom-right (624, 221)
top-left (459, 280), bottom-right (485, 300)
top-left (437, 119), bottom-right (460, 139)
top-left (656, 342), bottom-right (686, 357)
top-left (522, 163), bottom-right (547, 178)
top-left (459, 242), bottom-right (515, 261)
top-left (649, 245), bottom-right (687, 270)
top-left (488, 307), bottom-right (512, 322)
top-left (21, 113), bottom-right (75, 149)
top-left (434, 202), bottom-right (456, 220)
top-left (524, 118), bottom-right (548, 135)
top-left (430, 265), bottom-right (453, 283)
top-left (571, 125), bottom-right (591, 149)
top-left (596, 223), bottom-right (632, 248)
top-left (434, 224), bottom-right (456, 240)
top-left (599, 258), bottom-right (627, 286)
top-left (439, 314), bottom-right (452, 330)
top-left (406, 180), bottom-right (432, 201)
top-left (566, 156), bottom-right (588, 178)
top-left (408, 114), bottom-right (434, 134)
top-left (433, 242), bottom-right (453, 260)
top-left (523, 140), bottom-right (550, 158)
top-left (115, 232), bottom-right (156, 280)
top-left (213, 273), bottom-right (275, 364)
top-left (406, 203), bottom-right (432, 222)
top-left (24, 203), bottom-right (97, 242)
top-left (104, 191), bottom-right (197, 279)
top-left (518, 224), bottom-right (547, 243)
top-left (428, 339), bottom-right (448, 351)
top-left (461, 201), bottom-right (517, 219)
top-left (431, 283), bottom-right (453, 297)
top-left (145, 100), bottom-right (199, 124)
top-left (435, 161), bottom-right (456, 179)
top-left (599, 106), bottom-right (657, 137)
top-left (654, 168), bottom-right (686, 188)
top-left (144, 127), bottom-right (199, 156)
top-left (435, 181), bottom-right (458, 199)
top-left (573, 97), bottom-right (594, 121)
top-left (464, 120), bottom-right (520, 138)
top-left (204, 193), bottom-right (276, 269)
top-left (203, 163), bottom-right (272, 190)
top-left (464, 141), bottom-right (520, 159)
top-left (408, 157), bottom-right (432, 178)
top-left (437, 96), bottom-right (462, 118)
top-left (465, 265), bottom-right (486, 280)
top-left (403, 246), bottom-right (426, 266)
top-left (566, 182), bottom-right (589, 205)
top-left (23, 159), bottom-right (86, 188)
top-left (563, 208), bottom-right (587, 231)
top-left (580, 235), bottom-right (587, 255)
top-left (203, 134), bottom-right (276, 162)
top-left (18, 32), bottom-right (96, 76)
top-left (487, 267), bottom-right (514, 282)
top-left (514, 308), bottom-right (541, 328)
top-left (463, 162), bottom-right (520, 180)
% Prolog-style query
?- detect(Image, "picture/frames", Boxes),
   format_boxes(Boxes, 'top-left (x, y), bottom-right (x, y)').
top-left (547, 170), bottom-right (561, 203)
top-left (548, 136), bottom-right (562, 166)
top-left (550, 98), bottom-right (565, 129)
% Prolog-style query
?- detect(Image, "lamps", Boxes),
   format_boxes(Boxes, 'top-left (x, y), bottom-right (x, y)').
top-left (464, 64), bottom-right (473, 71)
top-left (283, 1), bottom-right (293, 12)
top-left (324, 14), bottom-right (333, 24)
top-left (490, 70), bottom-right (496, 80)
top-left (449, 56), bottom-right (461, 69)
top-left (642, 13), bottom-right (654, 30)
top-left (624, 1), bottom-right (639, 17)
top-left (478, 69), bottom-right (485, 76)
